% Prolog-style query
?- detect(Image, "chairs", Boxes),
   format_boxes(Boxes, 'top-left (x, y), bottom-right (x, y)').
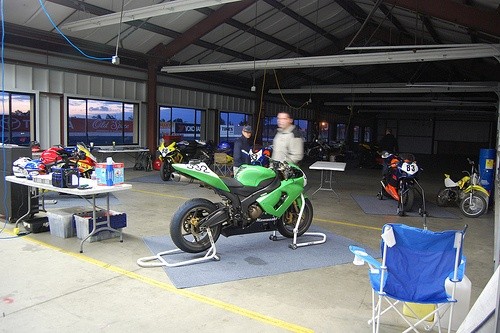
top-left (214, 153), bottom-right (233, 178)
top-left (349, 223), bottom-right (469, 333)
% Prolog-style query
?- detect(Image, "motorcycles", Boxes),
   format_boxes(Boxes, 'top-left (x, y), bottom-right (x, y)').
top-left (437, 156), bottom-right (490, 218)
top-left (159, 138), bottom-right (193, 181)
top-left (196, 139), bottom-right (234, 168)
top-left (169, 157), bottom-right (314, 253)
top-left (55, 142), bottom-right (97, 178)
top-left (378, 149), bottom-right (420, 211)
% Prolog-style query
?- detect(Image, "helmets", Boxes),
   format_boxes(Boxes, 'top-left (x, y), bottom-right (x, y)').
top-left (24, 159), bottom-right (48, 180)
top-left (12, 157), bottom-right (33, 178)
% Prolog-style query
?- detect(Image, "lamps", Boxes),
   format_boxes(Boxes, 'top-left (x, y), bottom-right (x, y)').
top-left (60, 0), bottom-right (500, 106)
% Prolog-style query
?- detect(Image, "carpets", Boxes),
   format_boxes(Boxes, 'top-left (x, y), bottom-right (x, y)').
top-left (351, 194), bottom-right (460, 219)
top-left (143, 225), bottom-right (381, 288)
top-left (125, 173), bottom-right (190, 185)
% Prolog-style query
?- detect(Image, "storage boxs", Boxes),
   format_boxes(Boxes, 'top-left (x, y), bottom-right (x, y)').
top-left (96, 163), bottom-right (124, 186)
top-left (22, 217), bottom-right (50, 233)
top-left (44, 206), bottom-right (127, 243)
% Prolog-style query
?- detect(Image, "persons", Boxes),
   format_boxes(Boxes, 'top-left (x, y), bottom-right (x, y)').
top-left (271, 111), bottom-right (303, 171)
top-left (233, 126), bottom-right (252, 177)
top-left (378, 128), bottom-right (399, 177)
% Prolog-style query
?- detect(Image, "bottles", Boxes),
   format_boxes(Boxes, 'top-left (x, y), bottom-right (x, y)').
top-left (434, 256), bottom-right (472, 331)
top-left (106, 156), bottom-right (114, 185)
top-left (403, 300), bottom-right (437, 321)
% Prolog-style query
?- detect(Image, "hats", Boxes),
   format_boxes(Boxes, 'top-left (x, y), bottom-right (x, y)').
top-left (242, 126), bottom-right (253, 133)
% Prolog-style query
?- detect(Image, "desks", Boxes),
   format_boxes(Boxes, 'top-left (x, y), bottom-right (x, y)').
top-left (309, 161), bottom-right (346, 199)
top-left (5, 176), bottom-right (133, 253)
top-left (97, 149), bottom-right (150, 172)
